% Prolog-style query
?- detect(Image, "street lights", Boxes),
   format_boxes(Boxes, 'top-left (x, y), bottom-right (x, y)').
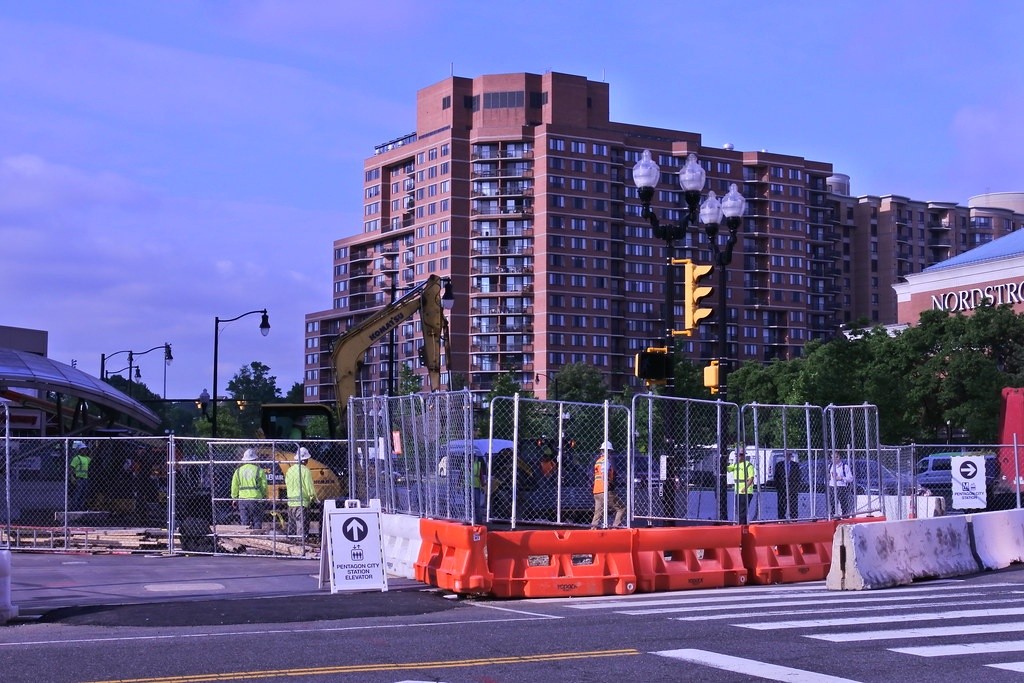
top-left (700, 182), bottom-right (747, 527)
top-left (127, 343), bottom-right (174, 428)
top-left (635, 149), bottom-right (708, 529)
top-left (196, 307), bottom-right (271, 440)
top-left (100, 350), bottom-right (136, 386)
top-left (105, 365), bottom-right (141, 386)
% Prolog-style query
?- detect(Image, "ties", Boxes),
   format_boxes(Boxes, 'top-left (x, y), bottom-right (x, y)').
top-left (787, 461), bottom-right (789, 474)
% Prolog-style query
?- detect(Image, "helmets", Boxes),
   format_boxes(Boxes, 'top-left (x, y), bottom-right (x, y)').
top-left (241, 449), bottom-right (258, 461)
top-left (78, 444), bottom-right (86, 451)
top-left (294, 447), bottom-right (311, 461)
top-left (734, 446), bottom-right (746, 454)
top-left (600, 440), bottom-right (614, 450)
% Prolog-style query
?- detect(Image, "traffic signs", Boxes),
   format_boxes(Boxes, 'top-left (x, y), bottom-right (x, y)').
top-left (948, 455), bottom-right (989, 512)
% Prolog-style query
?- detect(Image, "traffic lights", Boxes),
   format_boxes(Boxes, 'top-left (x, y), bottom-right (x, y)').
top-left (632, 350), bottom-right (673, 382)
top-left (680, 259), bottom-right (714, 332)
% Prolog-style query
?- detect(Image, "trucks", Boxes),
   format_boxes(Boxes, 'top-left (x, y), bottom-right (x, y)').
top-left (1000, 386), bottom-right (1024, 497)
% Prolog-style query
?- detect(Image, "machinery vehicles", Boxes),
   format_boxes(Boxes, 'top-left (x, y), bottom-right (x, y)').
top-left (222, 273), bottom-right (455, 522)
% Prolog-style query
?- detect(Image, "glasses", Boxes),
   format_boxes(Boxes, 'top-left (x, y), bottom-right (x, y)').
top-left (786, 452), bottom-right (792, 456)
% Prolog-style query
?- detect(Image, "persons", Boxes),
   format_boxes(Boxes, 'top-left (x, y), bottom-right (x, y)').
top-left (774, 452), bottom-right (804, 523)
top-left (231, 449), bottom-right (268, 529)
top-left (69, 444), bottom-right (91, 511)
top-left (285, 447), bottom-right (321, 542)
top-left (591, 441), bottom-right (626, 530)
top-left (457, 450), bottom-right (485, 527)
top-left (726, 448), bottom-right (754, 525)
top-left (824, 453), bottom-right (854, 521)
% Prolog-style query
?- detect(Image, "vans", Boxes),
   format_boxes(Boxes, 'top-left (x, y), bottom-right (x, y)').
top-left (907, 451), bottom-right (997, 488)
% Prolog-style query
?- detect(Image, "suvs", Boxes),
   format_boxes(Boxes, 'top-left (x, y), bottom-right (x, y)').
top-left (797, 459), bottom-right (932, 498)
